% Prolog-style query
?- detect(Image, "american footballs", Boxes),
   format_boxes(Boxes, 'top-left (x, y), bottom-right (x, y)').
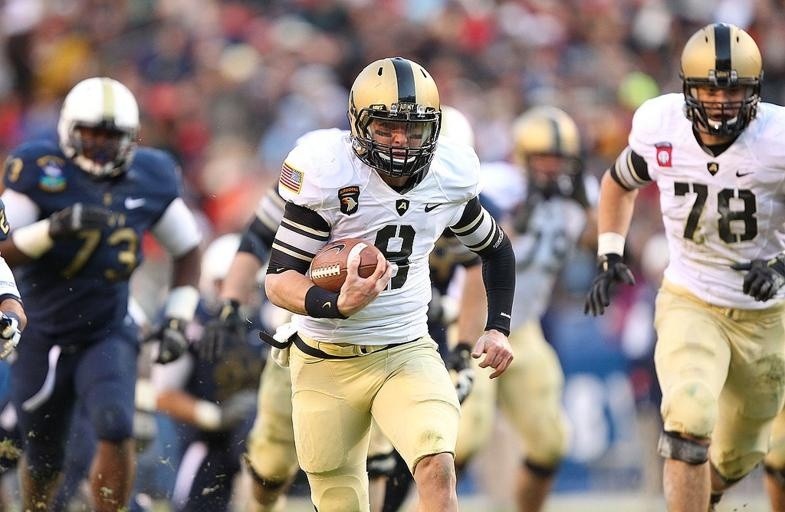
top-left (308, 241), bottom-right (388, 293)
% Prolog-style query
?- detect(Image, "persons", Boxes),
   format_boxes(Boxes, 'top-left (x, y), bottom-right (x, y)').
top-left (0, 1), bottom-right (784, 511)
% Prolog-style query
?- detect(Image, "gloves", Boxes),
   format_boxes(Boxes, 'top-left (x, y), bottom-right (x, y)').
top-left (582, 254), bottom-right (637, 318)
top-left (728, 251), bottom-right (785, 303)
top-left (0, 309), bottom-right (24, 359)
top-left (197, 300), bottom-right (246, 365)
top-left (140, 317), bottom-right (192, 365)
top-left (47, 203), bottom-right (113, 245)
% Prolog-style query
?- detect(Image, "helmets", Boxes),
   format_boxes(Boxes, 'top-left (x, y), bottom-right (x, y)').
top-left (677, 21), bottom-right (764, 136)
top-left (198, 234), bottom-right (271, 312)
top-left (511, 105), bottom-right (582, 196)
top-left (57, 75), bottom-right (140, 177)
top-left (347, 55), bottom-right (442, 179)
